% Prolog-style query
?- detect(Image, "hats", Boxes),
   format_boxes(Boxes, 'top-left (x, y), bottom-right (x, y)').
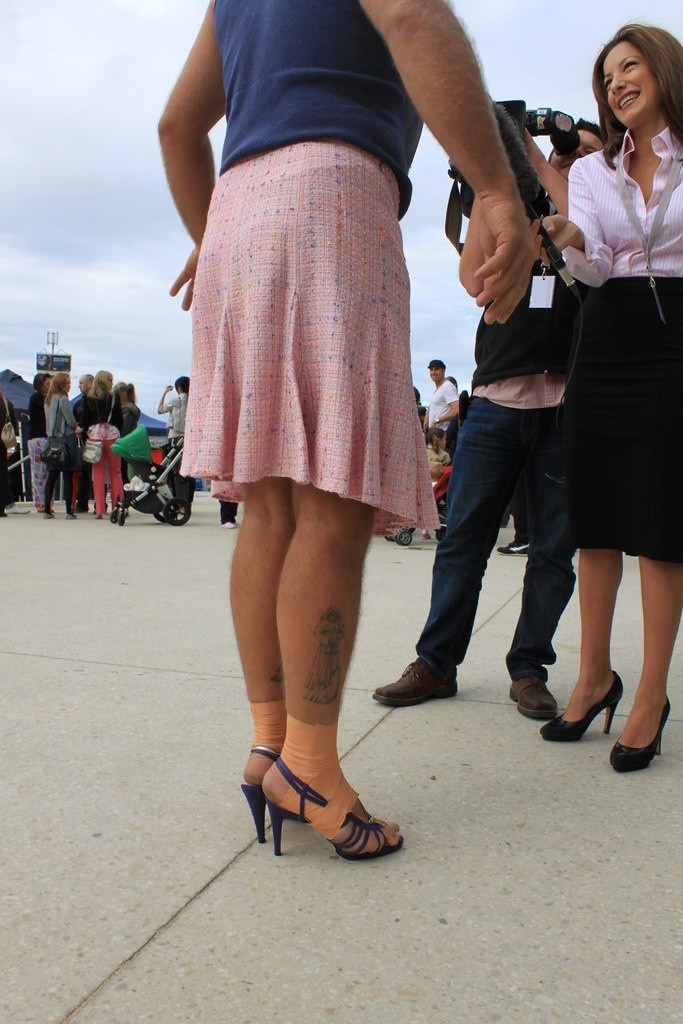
top-left (428, 360), bottom-right (446, 369)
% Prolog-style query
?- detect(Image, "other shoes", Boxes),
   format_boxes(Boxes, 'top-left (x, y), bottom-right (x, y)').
top-left (66, 513), bottom-right (76, 519)
top-left (44, 513), bottom-right (55, 519)
top-left (222, 522), bottom-right (235, 528)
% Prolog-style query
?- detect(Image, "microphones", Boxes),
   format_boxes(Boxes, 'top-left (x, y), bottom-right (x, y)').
top-left (487, 99), bottom-right (582, 300)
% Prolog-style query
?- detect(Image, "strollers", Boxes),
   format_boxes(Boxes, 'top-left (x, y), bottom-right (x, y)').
top-left (109, 422), bottom-right (197, 528)
top-left (385, 465), bottom-right (453, 546)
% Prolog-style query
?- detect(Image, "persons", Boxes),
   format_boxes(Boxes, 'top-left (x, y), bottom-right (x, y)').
top-left (0, 359), bottom-right (535, 555)
top-left (156, 0), bottom-right (540, 863)
top-left (372, 117), bottom-right (608, 722)
top-left (541, 24), bottom-right (683, 773)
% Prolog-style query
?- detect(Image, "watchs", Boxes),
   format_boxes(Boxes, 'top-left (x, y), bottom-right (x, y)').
top-left (435, 417), bottom-right (440, 423)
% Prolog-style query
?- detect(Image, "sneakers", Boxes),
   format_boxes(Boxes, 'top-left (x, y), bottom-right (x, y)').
top-left (497, 541), bottom-right (530, 556)
top-left (509, 676), bottom-right (556, 717)
top-left (373, 660), bottom-right (457, 704)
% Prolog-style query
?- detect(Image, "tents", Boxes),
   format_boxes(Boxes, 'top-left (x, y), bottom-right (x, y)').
top-left (0, 369), bottom-right (40, 501)
top-left (55, 392), bottom-right (169, 503)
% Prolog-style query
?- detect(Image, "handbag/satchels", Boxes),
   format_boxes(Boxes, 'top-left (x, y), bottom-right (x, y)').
top-left (1, 398), bottom-right (17, 448)
top-left (41, 441), bottom-right (70, 466)
top-left (81, 439), bottom-right (104, 463)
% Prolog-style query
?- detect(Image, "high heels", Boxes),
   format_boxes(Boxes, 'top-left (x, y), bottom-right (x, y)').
top-left (263, 757), bottom-right (403, 859)
top-left (610, 696), bottom-right (671, 772)
top-left (540, 670), bottom-right (623, 741)
top-left (240, 746), bottom-right (307, 844)
